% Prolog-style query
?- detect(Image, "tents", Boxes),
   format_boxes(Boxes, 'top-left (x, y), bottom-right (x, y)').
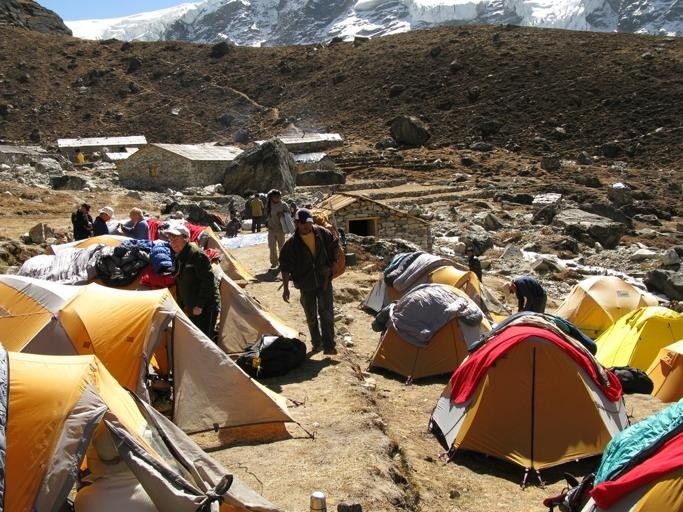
top-left (0, 341), bottom-right (284, 512)
top-left (16, 235), bottom-right (307, 354)
top-left (554, 275), bottom-right (683, 402)
top-left (369, 283), bottom-right (493, 386)
top-left (543, 401), bottom-right (683, 512)
top-left (429, 311), bottom-right (630, 489)
top-left (359, 263), bottom-right (511, 325)
top-left (105, 214), bottom-right (259, 285)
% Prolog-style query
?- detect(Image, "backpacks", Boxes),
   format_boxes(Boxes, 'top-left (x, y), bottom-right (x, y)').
top-left (233, 332), bottom-right (309, 380)
top-left (608, 366), bottom-right (655, 395)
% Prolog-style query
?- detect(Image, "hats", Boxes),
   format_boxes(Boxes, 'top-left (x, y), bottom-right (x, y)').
top-left (99, 207), bottom-right (114, 218)
top-left (294, 207), bottom-right (315, 224)
top-left (161, 224), bottom-right (191, 241)
top-left (81, 202), bottom-right (91, 210)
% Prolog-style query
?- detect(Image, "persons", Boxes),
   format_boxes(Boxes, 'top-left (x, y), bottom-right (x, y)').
top-left (278, 208), bottom-right (339, 355)
top-left (0, 274), bottom-right (315, 439)
top-left (157, 211), bottom-right (188, 242)
top-left (509, 276), bottom-right (547, 313)
top-left (92, 207), bottom-right (115, 236)
top-left (249, 193), bottom-right (265, 233)
top-left (264, 189), bottom-right (290, 268)
top-left (121, 207), bottom-right (149, 240)
top-left (466, 247), bottom-right (482, 283)
top-left (72, 203), bottom-right (93, 240)
top-left (163, 224), bottom-right (221, 340)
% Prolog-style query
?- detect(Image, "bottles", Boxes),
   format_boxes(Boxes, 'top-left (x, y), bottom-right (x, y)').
top-left (309, 492), bottom-right (326, 512)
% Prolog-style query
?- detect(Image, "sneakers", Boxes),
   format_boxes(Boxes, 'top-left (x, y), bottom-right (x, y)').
top-left (321, 345), bottom-right (339, 357)
